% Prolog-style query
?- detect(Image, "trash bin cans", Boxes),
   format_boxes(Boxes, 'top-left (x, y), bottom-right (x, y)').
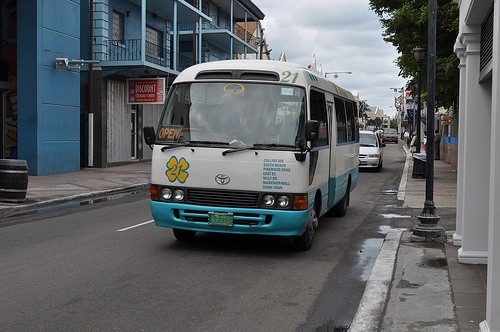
top-left (412, 153), bottom-right (426, 179)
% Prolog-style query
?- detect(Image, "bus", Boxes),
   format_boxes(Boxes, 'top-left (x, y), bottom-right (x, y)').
top-left (150, 59), bottom-right (361, 251)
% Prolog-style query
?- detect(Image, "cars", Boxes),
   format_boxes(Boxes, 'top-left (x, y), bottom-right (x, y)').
top-left (382, 128), bottom-right (397, 146)
top-left (359, 130), bottom-right (383, 172)
